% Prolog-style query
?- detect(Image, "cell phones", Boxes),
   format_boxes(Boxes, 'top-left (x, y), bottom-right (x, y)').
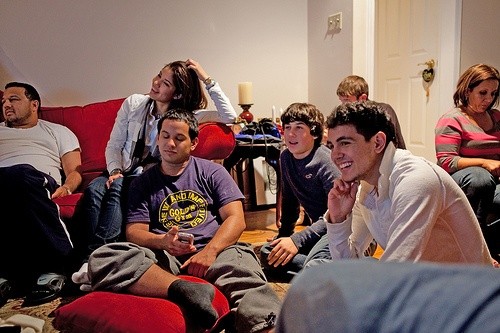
top-left (178, 232), bottom-right (194, 245)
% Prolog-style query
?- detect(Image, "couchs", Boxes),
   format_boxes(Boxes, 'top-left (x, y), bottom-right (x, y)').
top-left (39, 98), bottom-right (236, 219)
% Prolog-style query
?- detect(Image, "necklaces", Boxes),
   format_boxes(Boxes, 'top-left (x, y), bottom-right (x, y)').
top-left (153, 110), bottom-right (164, 117)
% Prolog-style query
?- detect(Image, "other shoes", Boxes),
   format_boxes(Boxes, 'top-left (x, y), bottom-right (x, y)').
top-left (22, 272), bottom-right (70, 303)
top-left (0, 277), bottom-right (13, 307)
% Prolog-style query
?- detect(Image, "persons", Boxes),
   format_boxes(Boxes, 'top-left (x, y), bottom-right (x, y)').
top-left (434, 62), bottom-right (500, 267)
top-left (322, 100), bottom-right (495, 267)
top-left (260, 102), bottom-right (377, 282)
top-left (71, 58), bottom-right (237, 290)
top-left (0, 81), bottom-right (83, 304)
top-left (86, 109), bottom-right (282, 333)
top-left (336, 74), bottom-right (407, 149)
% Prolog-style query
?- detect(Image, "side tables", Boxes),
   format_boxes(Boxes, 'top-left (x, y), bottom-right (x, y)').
top-left (236, 143), bottom-right (282, 229)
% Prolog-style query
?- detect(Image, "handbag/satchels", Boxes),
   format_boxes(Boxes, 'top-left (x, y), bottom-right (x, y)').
top-left (235, 117), bottom-right (281, 143)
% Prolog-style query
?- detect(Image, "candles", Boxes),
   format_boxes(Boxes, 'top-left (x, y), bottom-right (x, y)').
top-left (238, 81), bottom-right (253, 104)
top-left (272, 105), bottom-right (277, 123)
top-left (280, 108), bottom-right (283, 127)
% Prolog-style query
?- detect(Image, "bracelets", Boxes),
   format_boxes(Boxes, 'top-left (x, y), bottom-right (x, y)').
top-left (203, 75), bottom-right (212, 85)
top-left (63, 184), bottom-right (72, 195)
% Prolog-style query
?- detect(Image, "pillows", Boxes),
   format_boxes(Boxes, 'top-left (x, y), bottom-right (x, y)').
top-left (52, 275), bottom-right (230, 333)
